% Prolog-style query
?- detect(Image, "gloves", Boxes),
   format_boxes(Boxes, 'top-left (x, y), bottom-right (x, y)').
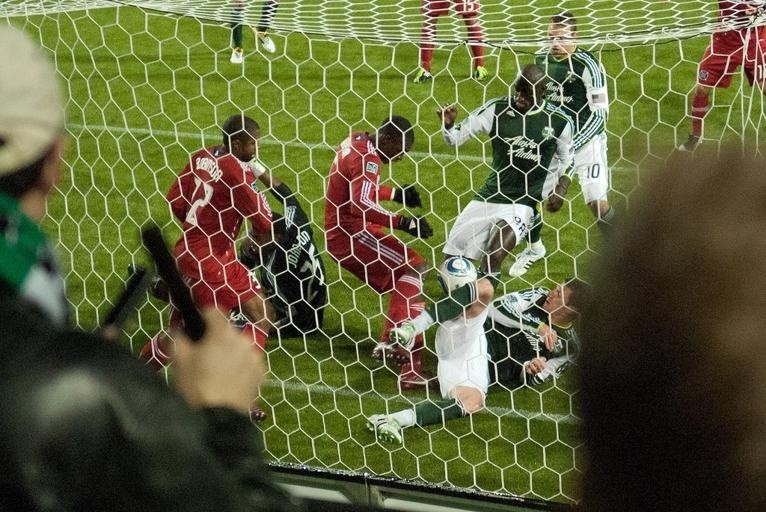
top-left (397, 214), bottom-right (434, 239)
top-left (391, 183), bottom-right (422, 209)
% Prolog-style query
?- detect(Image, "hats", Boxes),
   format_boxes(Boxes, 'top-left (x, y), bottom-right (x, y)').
top-left (0, 26), bottom-right (66, 178)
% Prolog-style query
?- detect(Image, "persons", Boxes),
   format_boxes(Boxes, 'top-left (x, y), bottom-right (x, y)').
top-left (503, 9), bottom-right (624, 278)
top-left (227, 1), bottom-right (281, 66)
top-left (0, 16), bottom-right (309, 511)
top-left (553, 137), bottom-right (766, 511)
top-left (364, 252), bottom-right (592, 448)
top-left (674, 0), bottom-right (766, 155)
top-left (129, 112), bottom-right (277, 423)
top-left (321, 111), bottom-right (442, 394)
top-left (434, 59), bottom-right (577, 292)
top-left (227, 157), bottom-right (328, 340)
top-left (408, 3), bottom-right (491, 88)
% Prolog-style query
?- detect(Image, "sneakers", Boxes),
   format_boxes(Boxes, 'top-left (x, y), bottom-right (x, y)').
top-left (678, 135), bottom-right (704, 154)
top-left (366, 413), bottom-right (403, 445)
top-left (475, 66), bottom-right (488, 82)
top-left (370, 343), bottom-right (420, 368)
top-left (228, 49), bottom-right (243, 64)
top-left (254, 30), bottom-right (276, 54)
top-left (398, 369), bottom-right (439, 391)
top-left (412, 68), bottom-right (432, 84)
top-left (389, 322), bottom-right (417, 351)
top-left (247, 404), bottom-right (269, 423)
top-left (507, 238), bottom-right (547, 278)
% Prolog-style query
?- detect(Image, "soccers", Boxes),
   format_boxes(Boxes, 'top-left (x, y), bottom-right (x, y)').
top-left (438, 256), bottom-right (477, 291)
